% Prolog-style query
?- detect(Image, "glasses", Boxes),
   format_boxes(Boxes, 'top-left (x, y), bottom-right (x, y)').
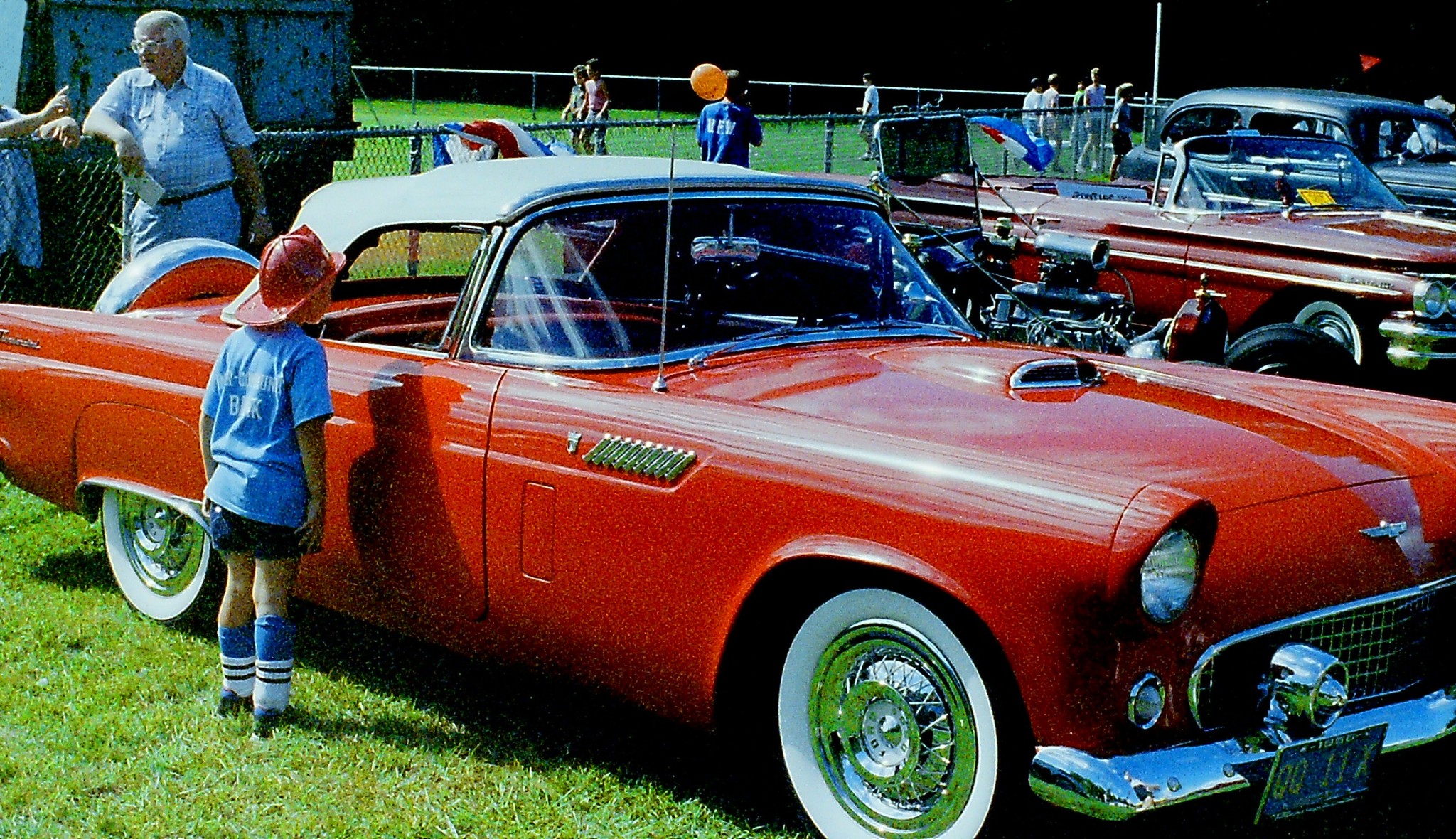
top-left (130, 38), bottom-right (182, 55)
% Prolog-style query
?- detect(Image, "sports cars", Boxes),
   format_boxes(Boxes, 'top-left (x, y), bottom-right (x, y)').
top-left (778, 111), bottom-right (1456, 395)
top-left (0, 157), bottom-right (1456, 839)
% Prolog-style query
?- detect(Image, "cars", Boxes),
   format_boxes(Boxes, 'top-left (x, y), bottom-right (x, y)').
top-left (1117, 86), bottom-right (1456, 222)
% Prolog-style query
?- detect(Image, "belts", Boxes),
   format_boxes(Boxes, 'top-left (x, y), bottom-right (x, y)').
top-left (158, 182), bottom-right (229, 207)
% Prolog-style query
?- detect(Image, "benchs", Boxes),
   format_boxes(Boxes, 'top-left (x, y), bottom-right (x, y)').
top-left (343, 312), bottom-right (665, 360)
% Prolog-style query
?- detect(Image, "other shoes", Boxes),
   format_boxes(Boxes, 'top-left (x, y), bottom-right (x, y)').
top-left (216, 690), bottom-right (254, 720)
top-left (252, 704), bottom-right (322, 738)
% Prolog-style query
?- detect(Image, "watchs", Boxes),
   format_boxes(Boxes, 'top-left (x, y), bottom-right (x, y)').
top-left (254, 207), bottom-right (270, 217)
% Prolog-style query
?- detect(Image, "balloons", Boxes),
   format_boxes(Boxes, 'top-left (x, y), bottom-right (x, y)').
top-left (690, 64), bottom-right (728, 101)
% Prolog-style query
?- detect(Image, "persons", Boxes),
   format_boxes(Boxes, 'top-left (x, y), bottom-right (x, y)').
top-left (198, 224), bottom-right (346, 744)
top-left (856, 73), bottom-right (880, 161)
top-left (1293, 95), bottom-right (1456, 159)
top-left (902, 217), bottom-right (1022, 333)
top-left (696, 74), bottom-right (763, 168)
top-left (1196, 112), bottom-right (1242, 127)
top-left (82, 10), bottom-right (274, 266)
top-left (1021, 67), bottom-right (1135, 182)
top-left (0, 85), bottom-right (81, 287)
top-left (561, 59), bottom-right (609, 156)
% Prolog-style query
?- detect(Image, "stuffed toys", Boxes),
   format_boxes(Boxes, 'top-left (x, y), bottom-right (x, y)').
top-left (1275, 177), bottom-right (1296, 207)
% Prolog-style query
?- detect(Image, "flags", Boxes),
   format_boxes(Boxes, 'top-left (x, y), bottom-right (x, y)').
top-left (966, 115), bottom-right (1054, 172)
top-left (431, 118), bottom-right (576, 167)
top-left (1360, 54), bottom-right (1381, 72)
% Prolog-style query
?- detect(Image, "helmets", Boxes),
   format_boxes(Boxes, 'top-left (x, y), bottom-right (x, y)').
top-left (234, 224), bottom-right (346, 326)
